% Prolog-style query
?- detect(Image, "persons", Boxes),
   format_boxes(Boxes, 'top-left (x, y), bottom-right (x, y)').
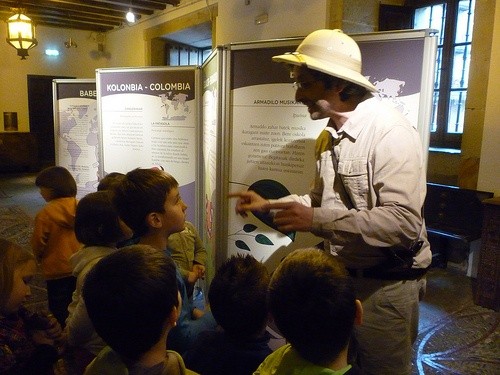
top-left (181, 252), bottom-right (289, 375)
top-left (226, 29), bottom-right (432, 375)
top-left (32, 166), bottom-right (84, 332)
top-left (81, 245), bottom-right (200, 375)
top-left (252, 248), bottom-right (363, 375)
top-left (0, 238), bottom-right (70, 375)
top-left (62, 168), bottom-right (218, 375)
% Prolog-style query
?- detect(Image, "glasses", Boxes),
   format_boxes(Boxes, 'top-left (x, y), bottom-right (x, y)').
top-left (292, 78), bottom-right (330, 90)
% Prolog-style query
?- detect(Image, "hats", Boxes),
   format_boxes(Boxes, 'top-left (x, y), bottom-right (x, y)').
top-left (272, 30), bottom-right (378, 94)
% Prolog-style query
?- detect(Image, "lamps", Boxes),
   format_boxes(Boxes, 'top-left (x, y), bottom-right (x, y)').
top-left (64, 35), bottom-right (77, 49)
top-left (5, 2), bottom-right (38, 59)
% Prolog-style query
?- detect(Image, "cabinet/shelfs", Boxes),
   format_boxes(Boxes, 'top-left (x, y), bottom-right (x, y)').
top-left (476, 198), bottom-right (500, 311)
top-left (422, 183), bottom-right (494, 268)
